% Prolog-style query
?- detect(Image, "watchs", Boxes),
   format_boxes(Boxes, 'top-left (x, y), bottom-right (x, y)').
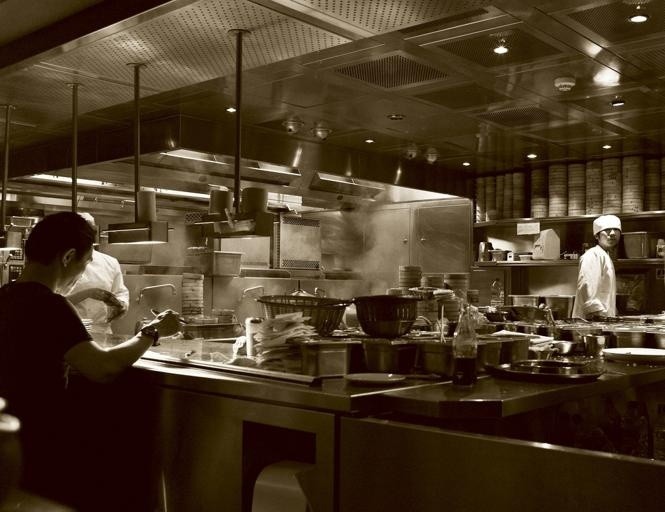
top-left (137, 322), bottom-right (161, 347)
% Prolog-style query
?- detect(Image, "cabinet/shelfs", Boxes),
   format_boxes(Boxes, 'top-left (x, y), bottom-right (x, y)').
top-left (472, 210), bottom-right (665, 266)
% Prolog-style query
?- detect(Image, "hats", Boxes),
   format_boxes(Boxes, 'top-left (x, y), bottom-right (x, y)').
top-left (76, 211), bottom-right (97, 230)
top-left (593, 215), bottom-right (621, 236)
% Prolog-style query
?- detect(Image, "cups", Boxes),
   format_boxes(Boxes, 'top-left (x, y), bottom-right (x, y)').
top-left (583, 334), bottom-right (605, 356)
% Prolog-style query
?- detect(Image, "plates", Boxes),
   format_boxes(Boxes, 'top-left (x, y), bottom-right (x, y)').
top-left (599, 347), bottom-right (665, 363)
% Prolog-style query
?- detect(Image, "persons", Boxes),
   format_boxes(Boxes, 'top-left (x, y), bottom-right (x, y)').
top-left (571, 214), bottom-right (623, 321)
top-left (53, 210), bottom-right (131, 348)
top-left (0, 210), bottom-right (183, 512)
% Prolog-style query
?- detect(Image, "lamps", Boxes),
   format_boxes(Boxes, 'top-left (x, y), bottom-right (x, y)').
top-left (99, 62), bottom-right (174, 245)
top-left (201, 28), bottom-right (274, 239)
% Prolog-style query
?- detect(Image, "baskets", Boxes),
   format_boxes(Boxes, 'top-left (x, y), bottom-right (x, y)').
top-left (253, 293), bottom-right (350, 335)
top-left (354, 296), bottom-right (425, 335)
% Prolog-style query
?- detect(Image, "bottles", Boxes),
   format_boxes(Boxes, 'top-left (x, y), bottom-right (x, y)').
top-left (245, 317), bottom-right (265, 362)
top-left (490, 278), bottom-right (504, 306)
top-left (452, 304), bottom-right (478, 388)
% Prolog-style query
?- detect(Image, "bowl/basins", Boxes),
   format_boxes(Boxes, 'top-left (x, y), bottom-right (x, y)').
top-left (552, 340), bottom-right (571, 355)
